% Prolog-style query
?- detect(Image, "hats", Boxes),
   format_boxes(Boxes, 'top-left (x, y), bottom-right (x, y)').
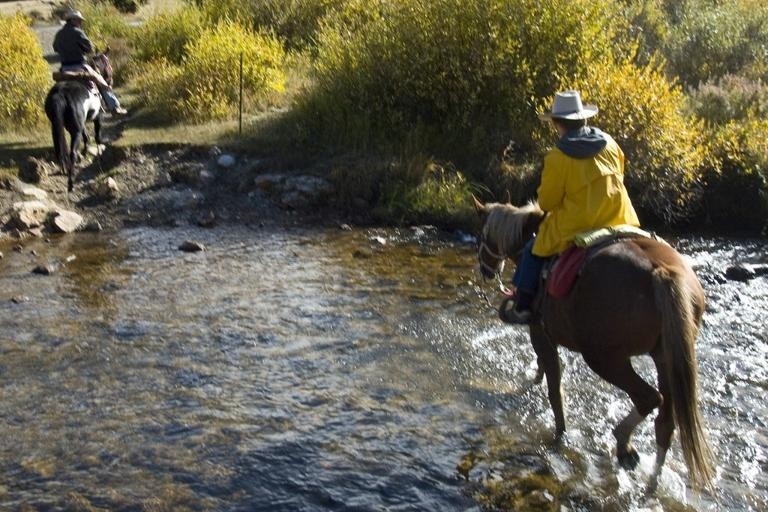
top-left (63, 10), bottom-right (87, 23)
top-left (537, 90), bottom-right (600, 123)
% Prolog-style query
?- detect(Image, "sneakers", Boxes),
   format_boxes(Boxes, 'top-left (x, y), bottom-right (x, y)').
top-left (111, 106), bottom-right (128, 116)
top-left (504, 303), bottom-right (533, 324)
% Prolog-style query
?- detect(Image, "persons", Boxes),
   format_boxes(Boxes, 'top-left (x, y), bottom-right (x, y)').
top-left (495, 89), bottom-right (645, 322)
top-left (49, 11), bottom-right (128, 119)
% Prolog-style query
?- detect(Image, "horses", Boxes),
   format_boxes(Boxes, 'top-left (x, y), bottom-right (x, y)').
top-left (470, 189), bottom-right (723, 500)
top-left (43, 45), bottom-right (114, 194)
